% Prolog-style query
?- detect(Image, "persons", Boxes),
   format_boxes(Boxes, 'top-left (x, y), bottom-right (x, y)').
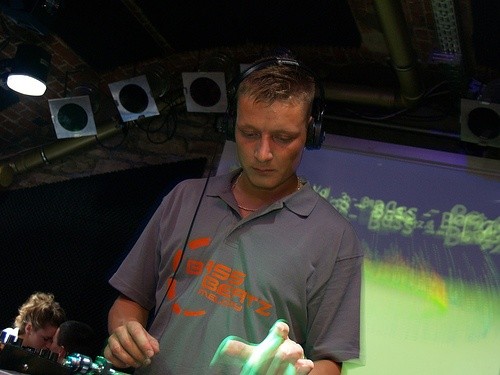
top-left (50, 320), bottom-right (92, 364)
top-left (0, 292), bottom-right (67, 351)
top-left (104, 56), bottom-right (365, 375)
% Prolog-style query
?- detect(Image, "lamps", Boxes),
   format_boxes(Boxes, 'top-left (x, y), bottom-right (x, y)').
top-left (183, 49), bottom-right (244, 113)
top-left (7, 43), bottom-right (52, 97)
top-left (47, 68), bottom-right (102, 139)
top-left (459, 80), bottom-right (500, 147)
top-left (108, 57), bottom-right (180, 123)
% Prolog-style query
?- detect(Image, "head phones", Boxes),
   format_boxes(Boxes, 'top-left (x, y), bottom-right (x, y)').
top-left (217, 57), bottom-right (327, 151)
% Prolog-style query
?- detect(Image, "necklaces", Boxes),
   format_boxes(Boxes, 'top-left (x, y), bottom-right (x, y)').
top-left (229, 169), bottom-right (302, 213)
